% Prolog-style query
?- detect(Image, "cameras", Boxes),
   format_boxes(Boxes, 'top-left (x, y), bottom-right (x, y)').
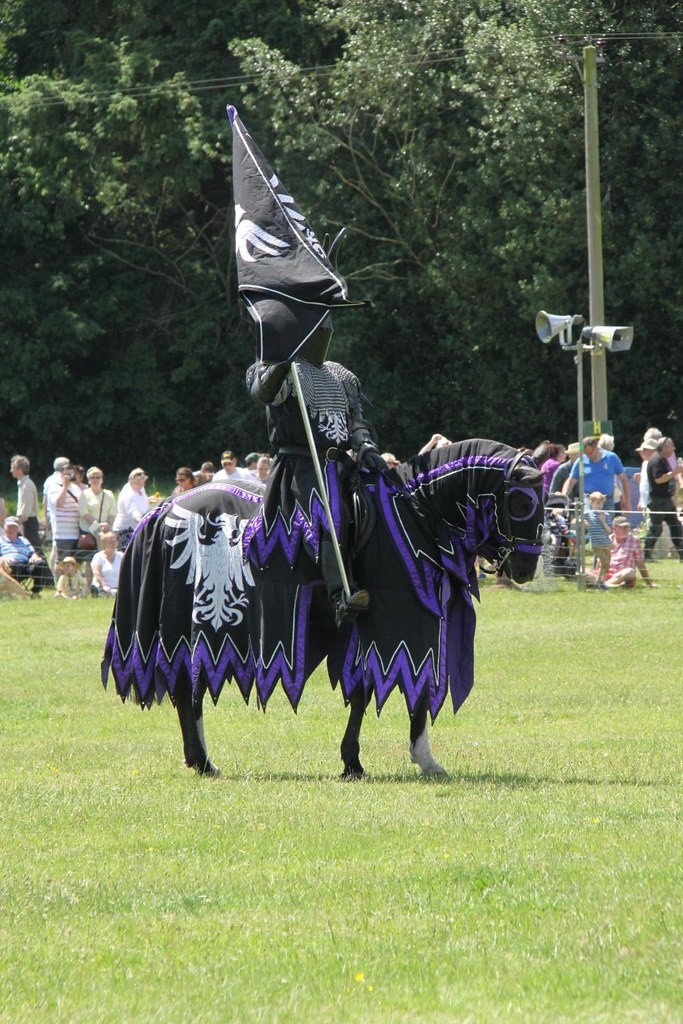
top-left (70, 474), bottom-right (77, 481)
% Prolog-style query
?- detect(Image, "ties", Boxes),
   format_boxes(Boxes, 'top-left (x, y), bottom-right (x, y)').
top-left (67, 577), bottom-right (72, 588)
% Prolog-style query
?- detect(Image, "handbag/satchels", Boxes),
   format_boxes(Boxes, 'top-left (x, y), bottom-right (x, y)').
top-left (75, 533), bottom-right (96, 548)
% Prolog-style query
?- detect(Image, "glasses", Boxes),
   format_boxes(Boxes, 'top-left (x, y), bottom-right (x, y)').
top-left (133, 472), bottom-right (148, 478)
top-left (89, 476), bottom-right (102, 480)
top-left (178, 478), bottom-right (189, 483)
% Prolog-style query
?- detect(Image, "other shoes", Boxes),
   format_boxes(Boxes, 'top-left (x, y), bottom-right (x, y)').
top-left (331, 590), bottom-right (371, 633)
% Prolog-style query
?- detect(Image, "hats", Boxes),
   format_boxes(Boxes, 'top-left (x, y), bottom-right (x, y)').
top-left (564, 442), bottom-right (581, 454)
top-left (641, 438), bottom-right (659, 450)
top-left (57, 556), bottom-right (80, 569)
top-left (635, 442), bottom-right (644, 451)
top-left (611, 515), bottom-right (630, 528)
top-left (219, 450), bottom-right (237, 464)
top-left (3, 515), bottom-right (21, 529)
top-left (244, 452), bottom-right (262, 464)
top-left (533, 440), bottom-right (550, 462)
top-left (381, 453), bottom-right (401, 466)
top-left (581, 434), bottom-right (601, 446)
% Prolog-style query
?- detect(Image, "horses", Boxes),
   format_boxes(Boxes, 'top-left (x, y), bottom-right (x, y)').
top-left (102, 438), bottom-right (549, 782)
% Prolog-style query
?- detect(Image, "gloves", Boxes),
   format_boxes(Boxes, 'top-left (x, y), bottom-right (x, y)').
top-left (358, 441), bottom-right (387, 473)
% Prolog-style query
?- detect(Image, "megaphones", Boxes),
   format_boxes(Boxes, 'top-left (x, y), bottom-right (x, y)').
top-left (535, 311), bottom-right (583, 344)
top-left (582, 326), bottom-right (633, 352)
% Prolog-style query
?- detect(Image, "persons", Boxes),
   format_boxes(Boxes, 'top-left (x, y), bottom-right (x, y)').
top-left (0, 450), bottom-right (277, 601)
top-left (380, 428), bottom-right (683, 589)
top-left (246, 313), bottom-right (388, 631)
